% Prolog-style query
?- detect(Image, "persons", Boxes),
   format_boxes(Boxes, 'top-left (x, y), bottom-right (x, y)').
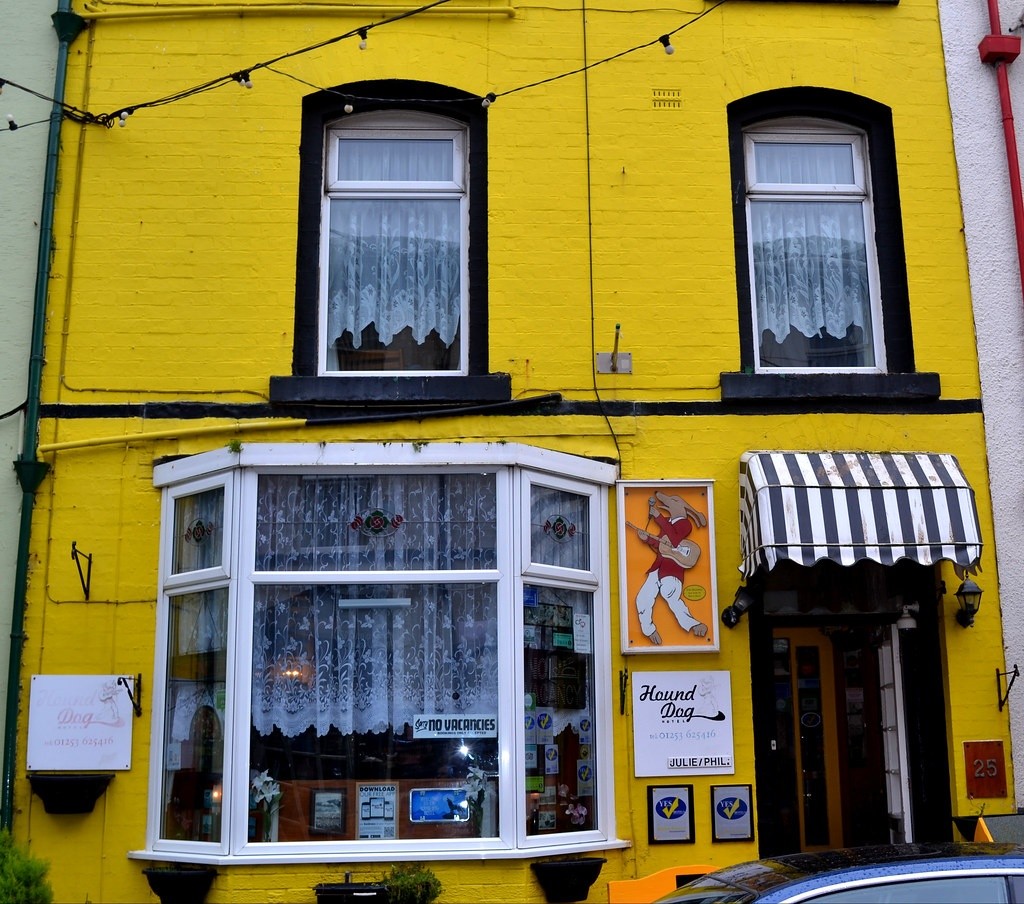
top-left (759, 739), bottom-right (808, 856)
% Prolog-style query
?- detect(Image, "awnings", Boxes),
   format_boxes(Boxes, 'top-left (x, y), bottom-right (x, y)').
top-left (739, 449), bottom-right (983, 589)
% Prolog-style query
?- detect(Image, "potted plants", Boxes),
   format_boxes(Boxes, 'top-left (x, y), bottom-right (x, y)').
top-left (530, 852), bottom-right (607, 904)
top-left (142, 859), bottom-right (217, 904)
top-left (312, 863), bottom-right (444, 904)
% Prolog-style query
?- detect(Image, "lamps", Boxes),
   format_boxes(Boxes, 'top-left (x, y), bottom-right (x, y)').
top-left (952, 570), bottom-right (984, 629)
top-left (722, 581), bottom-right (763, 628)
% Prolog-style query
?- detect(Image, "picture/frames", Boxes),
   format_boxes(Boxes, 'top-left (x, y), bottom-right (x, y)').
top-left (646, 784), bottom-right (696, 846)
top-left (710, 784), bottom-right (756, 843)
top-left (308, 787), bottom-right (347, 837)
top-left (407, 786), bottom-right (473, 824)
top-left (355, 781), bottom-right (400, 840)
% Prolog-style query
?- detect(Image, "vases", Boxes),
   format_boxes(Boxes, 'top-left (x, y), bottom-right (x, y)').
top-left (26, 774), bottom-right (115, 816)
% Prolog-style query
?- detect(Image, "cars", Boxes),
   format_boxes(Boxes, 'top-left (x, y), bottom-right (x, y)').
top-left (651, 842), bottom-right (1024, 904)
